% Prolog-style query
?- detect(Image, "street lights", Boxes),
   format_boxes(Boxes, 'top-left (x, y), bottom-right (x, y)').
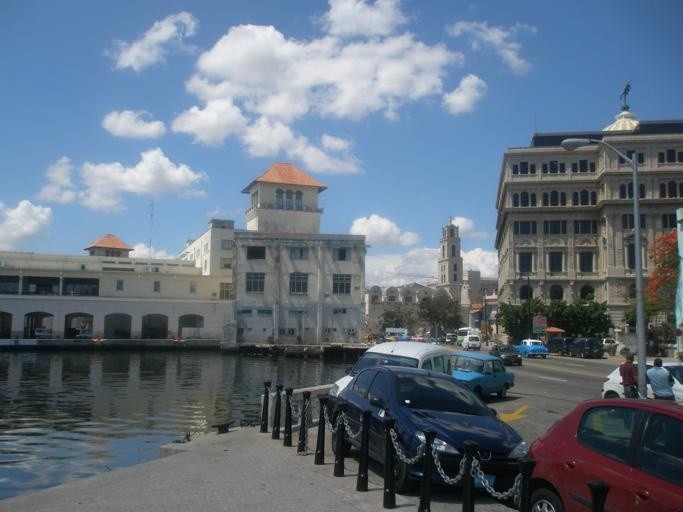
top-left (561, 137), bottom-right (646, 400)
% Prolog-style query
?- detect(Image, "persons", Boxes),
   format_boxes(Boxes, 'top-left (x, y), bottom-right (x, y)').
top-left (618, 353), bottom-right (637, 429)
top-left (645, 357), bottom-right (675, 401)
